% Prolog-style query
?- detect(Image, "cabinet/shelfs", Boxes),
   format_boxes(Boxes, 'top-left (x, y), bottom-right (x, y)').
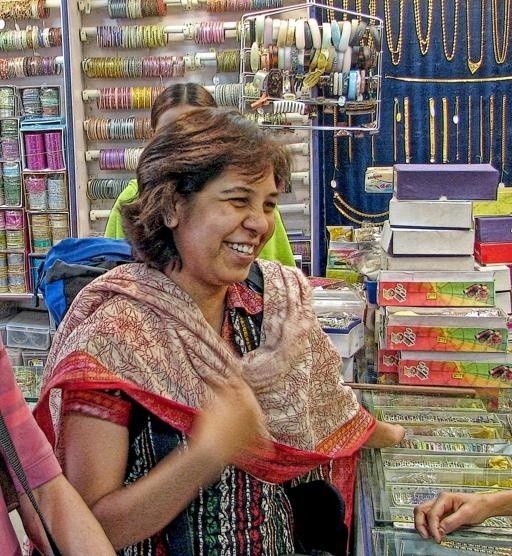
top-left (0, 1), bottom-right (75, 301)
top-left (66, 0), bottom-right (319, 277)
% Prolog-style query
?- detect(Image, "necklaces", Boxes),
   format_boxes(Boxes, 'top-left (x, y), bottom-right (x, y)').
top-left (491, 0), bottom-right (511, 63)
top-left (466, 0), bottom-right (485, 74)
top-left (356, 0), bottom-right (376, 46)
top-left (413, 0), bottom-right (434, 56)
top-left (326, 0), bottom-right (348, 22)
top-left (333, 202), bottom-right (384, 227)
top-left (440, 539), bottom-right (512, 556)
top-left (441, 0), bottom-right (458, 62)
top-left (333, 95), bottom-right (508, 170)
top-left (334, 191), bottom-right (389, 218)
top-left (384, 0), bottom-right (403, 65)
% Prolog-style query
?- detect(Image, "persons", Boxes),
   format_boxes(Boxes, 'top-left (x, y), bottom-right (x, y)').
top-left (28, 105), bottom-right (406, 556)
top-left (413, 492), bottom-right (511, 544)
top-left (104, 82), bottom-right (296, 267)
top-left (0, 337), bottom-right (117, 556)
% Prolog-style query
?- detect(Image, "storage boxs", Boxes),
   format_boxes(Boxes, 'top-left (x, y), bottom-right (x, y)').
top-left (0, 253), bottom-right (29, 296)
top-left (354, 227), bottom-right (381, 250)
top-left (20, 352), bottom-right (48, 367)
top-left (20, 125), bottom-right (68, 172)
top-left (377, 326), bottom-right (398, 373)
top-left (10, 364), bottom-right (44, 404)
top-left (26, 210), bottom-right (69, 255)
top-left (396, 349), bottom-right (512, 390)
top-left (0, 162), bottom-right (23, 208)
top-left (309, 288), bottom-right (365, 320)
top-left (3, 347), bottom-right (22, 367)
top-left (475, 265), bottom-right (512, 293)
top-left (0, 84), bottom-right (15, 121)
top-left (288, 235), bottom-right (312, 262)
top-left (494, 291), bottom-right (512, 316)
top-left (388, 197), bottom-right (475, 230)
top-left (0, 118), bottom-right (21, 163)
top-left (378, 226), bottom-right (476, 258)
top-left (471, 187), bottom-right (511, 218)
top-left (6, 309), bottom-right (51, 352)
top-left (391, 163), bottom-right (499, 201)
top-left (0, 314), bottom-right (16, 346)
top-left (474, 217), bottom-right (512, 244)
top-left (377, 272), bottom-right (495, 309)
top-left (324, 225), bottom-right (359, 250)
top-left (21, 171), bottom-right (70, 212)
top-left (475, 240), bottom-right (512, 264)
top-left (0, 209), bottom-right (26, 253)
top-left (383, 304), bottom-right (509, 354)
top-left (16, 85), bottom-right (64, 121)
top-left (376, 252), bottom-right (476, 271)
top-left (316, 315), bottom-right (366, 359)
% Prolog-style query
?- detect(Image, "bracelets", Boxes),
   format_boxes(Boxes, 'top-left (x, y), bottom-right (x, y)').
top-left (96, 24), bottom-right (169, 49)
top-left (392, 512), bottom-right (414, 522)
top-left (0, 210), bottom-right (24, 250)
top-left (97, 86), bottom-right (166, 110)
top-left (0, 0), bottom-right (50, 20)
top-left (193, 21), bottom-right (224, 44)
top-left (398, 440), bottom-right (493, 453)
top-left (22, 88), bottom-right (59, 117)
top-left (7, 323), bottom-right (29, 346)
top-left (243, 113), bottom-right (286, 125)
top-left (406, 426), bottom-right (471, 437)
top-left (107, 0), bottom-right (166, 20)
top-left (100, 147), bottom-right (145, 171)
top-left (206, 0), bottom-right (282, 14)
top-left (0, 162), bottom-right (20, 206)
top-left (389, 487), bottom-right (436, 506)
top-left (15, 371), bottom-right (35, 395)
top-left (0, 25), bottom-right (62, 52)
top-left (24, 134), bottom-right (45, 170)
top-left (214, 83), bottom-right (239, 106)
top-left (217, 50), bottom-right (239, 72)
top-left (87, 118), bottom-right (154, 141)
top-left (0, 56), bottom-right (64, 81)
top-left (484, 517), bottom-right (510, 527)
top-left (1, 120), bottom-right (17, 160)
top-left (26, 174), bottom-right (66, 210)
top-left (84, 56), bottom-right (185, 79)
top-left (0, 88), bottom-right (15, 117)
top-left (381, 411), bottom-right (488, 423)
top-left (392, 473), bottom-right (437, 484)
top-left (237, 21), bottom-right (241, 43)
top-left (383, 457), bottom-right (470, 470)
top-left (31, 214), bottom-right (69, 253)
top-left (0, 254), bottom-right (26, 294)
top-left (86, 179), bottom-right (129, 201)
top-left (44, 133), bottom-right (64, 170)
top-left (29, 325), bottom-right (51, 348)
top-left (33, 259), bottom-right (44, 293)
top-left (246, 16), bottom-right (381, 100)
